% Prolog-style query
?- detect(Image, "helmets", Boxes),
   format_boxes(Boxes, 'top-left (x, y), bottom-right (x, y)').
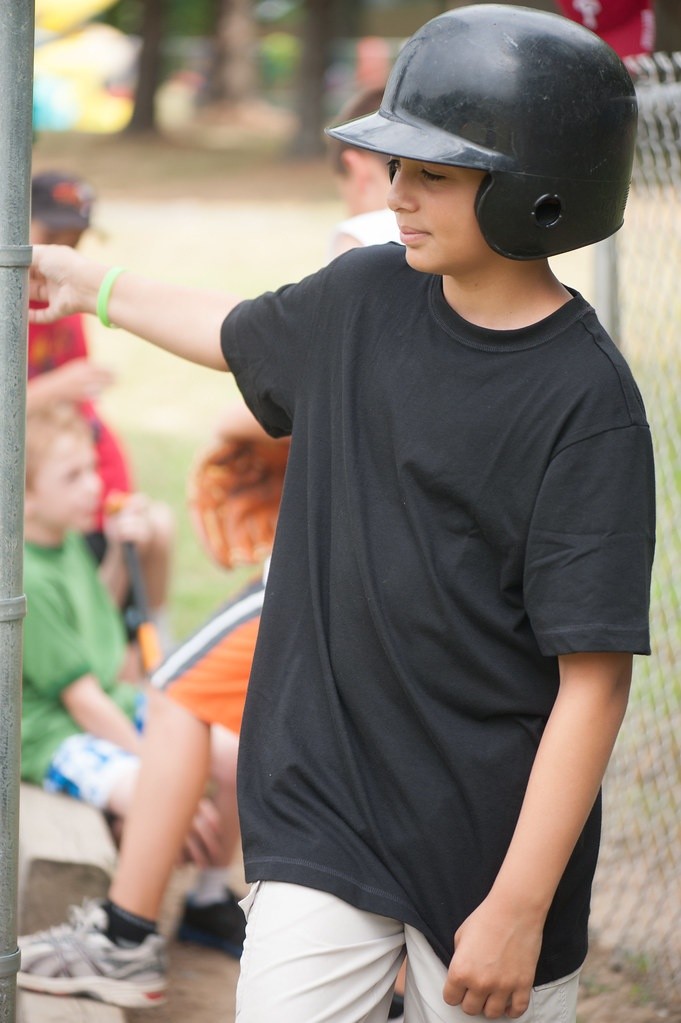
top-left (325, 3), bottom-right (637, 261)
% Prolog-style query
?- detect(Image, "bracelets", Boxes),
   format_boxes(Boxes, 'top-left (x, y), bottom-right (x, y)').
top-left (96, 266), bottom-right (126, 329)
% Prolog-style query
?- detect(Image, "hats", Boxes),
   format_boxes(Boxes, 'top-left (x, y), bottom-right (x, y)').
top-left (31, 169), bottom-right (93, 233)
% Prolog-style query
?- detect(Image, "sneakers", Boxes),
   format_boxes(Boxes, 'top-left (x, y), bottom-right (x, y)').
top-left (16, 896), bottom-right (170, 1009)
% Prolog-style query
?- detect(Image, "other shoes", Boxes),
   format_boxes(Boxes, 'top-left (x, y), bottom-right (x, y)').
top-left (184, 885), bottom-right (246, 956)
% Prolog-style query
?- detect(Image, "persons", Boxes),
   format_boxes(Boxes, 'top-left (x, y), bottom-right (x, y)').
top-left (325, 86), bottom-right (405, 258)
top-left (30, 167), bottom-right (172, 616)
top-left (15, 424), bottom-right (293, 1009)
top-left (29, 4), bottom-right (658, 1023)
top-left (25, 400), bottom-right (246, 958)
